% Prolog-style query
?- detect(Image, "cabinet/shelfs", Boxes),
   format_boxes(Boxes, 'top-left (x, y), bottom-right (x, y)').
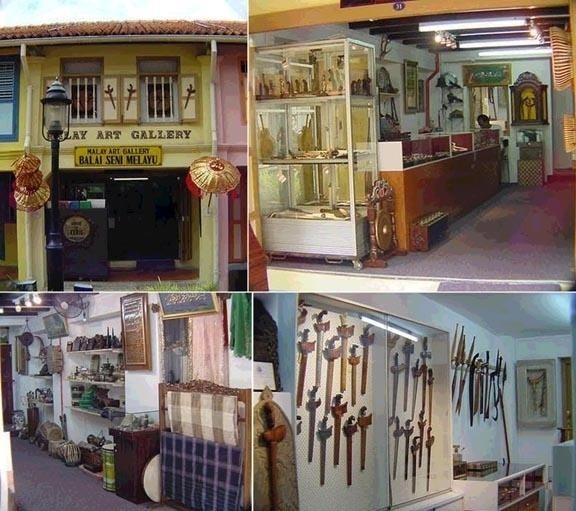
top-left (28, 354), bottom-right (52, 405)
top-left (62, 346), bottom-right (125, 419)
top-left (252, 36), bottom-right (383, 268)
top-left (108, 422), bottom-right (170, 507)
top-left (456, 462), bottom-right (547, 511)
top-left (376, 56), bottom-right (403, 98)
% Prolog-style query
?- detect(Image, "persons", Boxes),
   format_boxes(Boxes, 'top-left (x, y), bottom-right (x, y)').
top-left (476, 114), bottom-right (504, 152)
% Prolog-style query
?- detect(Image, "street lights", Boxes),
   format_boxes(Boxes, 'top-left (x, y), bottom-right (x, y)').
top-left (39, 75), bottom-right (73, 293)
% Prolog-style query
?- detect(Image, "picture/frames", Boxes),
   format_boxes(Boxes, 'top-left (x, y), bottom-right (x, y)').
top-left (462, 64), bottom-right (513, 88)
top-left (404, 59), bottom-right (421, 115)
top-left (119, 294), bottom-right (152, 372)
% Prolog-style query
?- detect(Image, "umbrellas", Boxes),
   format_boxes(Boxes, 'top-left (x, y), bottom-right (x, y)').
top-left (190, 155), bottom-right (242, 215)
top-left (11, 148), bottom-right (51, 212)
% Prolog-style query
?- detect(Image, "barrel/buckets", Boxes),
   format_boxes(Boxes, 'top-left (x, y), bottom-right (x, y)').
top-left (15, 280), bottom-right (37, 291)
top-left (102, 442), bottom-right (116, 491)
